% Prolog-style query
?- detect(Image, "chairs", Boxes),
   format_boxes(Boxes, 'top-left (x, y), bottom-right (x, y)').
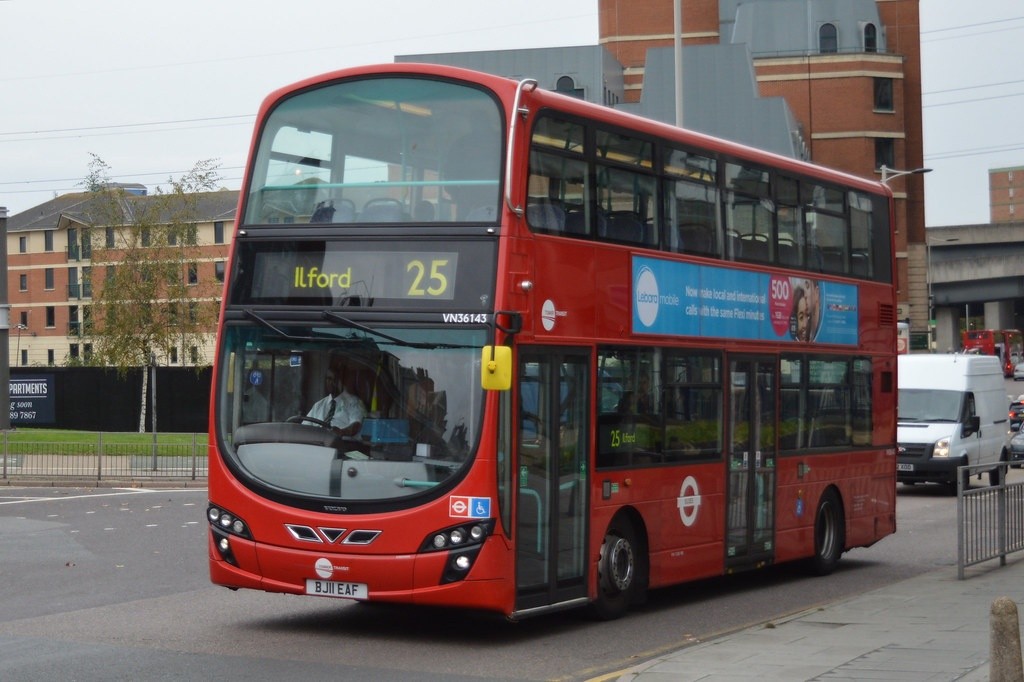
top-left (777, 238), bottom-right (799, 265)
top-left (605, 211), bottom-right (644, 242)
top-left (822, 246), bottom-right (843, 273)
top-left (560, 202), bottom-right (607, 237)
top-left (808, 243), bottom-right (825, 271)
top-left (852, 250), bottom-right (869, 278)
top-left (659, 222), bottom-right (712, 253)
top-left (309, 198), bottom-right (356, 223)
top-left (711, 227), bottom-right (743, 258)
top-left (527, 203), bottom-right (567, 230)
top-left (736, 232), bottom-right (768, 262)
top-left (356, 198), bottom-right (404, 222)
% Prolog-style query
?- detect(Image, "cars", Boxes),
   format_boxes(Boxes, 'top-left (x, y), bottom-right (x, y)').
top-left (1014, 361), bottom-right (1024, 381)
top-left (1009, 394), bottom-right (1024, 427)
top-left (1008, 423), bottom-right (1024, 469)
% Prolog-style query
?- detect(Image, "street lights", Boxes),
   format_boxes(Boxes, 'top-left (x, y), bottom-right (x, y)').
top-left (12, 323), bottom-right (29, 367)
top-left (927, 236), bottom-right (961, 355)
top-left (880, 165), bottom-right (933, 186)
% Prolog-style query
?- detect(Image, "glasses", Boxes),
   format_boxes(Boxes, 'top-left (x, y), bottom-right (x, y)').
top-left (325, 377), bottom-right (337, 382)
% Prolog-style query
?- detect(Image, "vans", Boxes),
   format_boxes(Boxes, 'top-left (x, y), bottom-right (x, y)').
top-left (895, 354), bottom-right (1013, 493)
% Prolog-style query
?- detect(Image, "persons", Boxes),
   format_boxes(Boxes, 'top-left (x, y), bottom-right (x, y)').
top-left (300, 364), bottom-right (367, 440)
top-left (792, 279), bottom-right (821, 343)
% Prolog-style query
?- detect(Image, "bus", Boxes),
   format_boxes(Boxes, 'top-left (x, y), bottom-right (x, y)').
top-left (962, 329), bottom-right (1022, 379)
top-left (206, 62), bottom-right (900, 626)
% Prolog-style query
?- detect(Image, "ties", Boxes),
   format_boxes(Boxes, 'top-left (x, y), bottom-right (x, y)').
top-left (323, 399), bottom-right (336, 428)
